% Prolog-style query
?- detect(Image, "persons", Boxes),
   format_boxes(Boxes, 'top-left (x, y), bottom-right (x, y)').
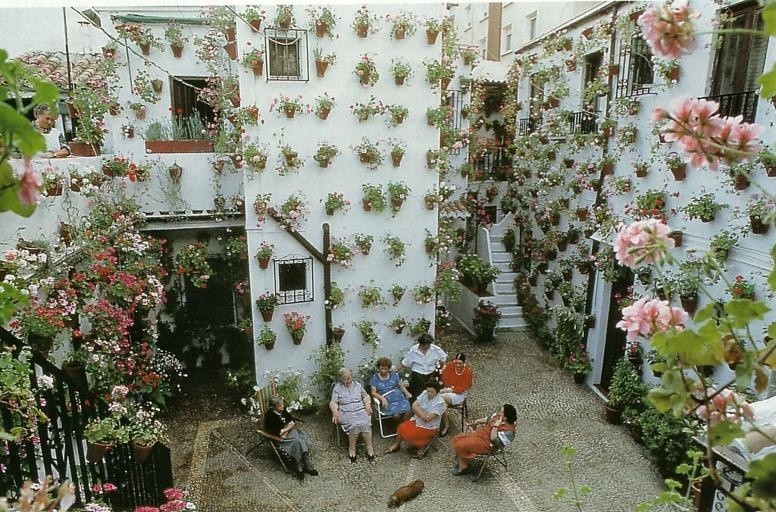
top-left (449, 403), bottom-right (516, 472)
top-left (438, 352), bottom-right (473, 422)
top-left (329, 366), bottom-right (375, 462)
top-left (24, 104), bottom-right (71, 158)
top-left (396, 335), bottom-right (447, 403)
top-left (368, 357), bottom-right (412, 428)
top-left (267, 393), bottom-right (318, 481)
top-left (385, 380), bottom-right (445, 458)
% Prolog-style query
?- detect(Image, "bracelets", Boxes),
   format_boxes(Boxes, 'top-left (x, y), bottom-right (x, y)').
top-left (493, 425), bottom-right (500, 428)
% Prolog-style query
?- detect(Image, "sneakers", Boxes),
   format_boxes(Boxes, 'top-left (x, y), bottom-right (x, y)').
top-left (367, 452), bottom-right (375, 462)
top-left (297, 470), bottom-right (304, 480)
top-left (349, 454), bottom-right (356, 462)
top-left (384, 447), bottom-right (400, 453)
top-left (304, 465), bottom-right (318, 475)
top-left (452, 461), bottom-right (470, 475)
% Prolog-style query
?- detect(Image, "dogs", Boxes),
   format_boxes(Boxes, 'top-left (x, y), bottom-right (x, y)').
top-left (386, 478), bottom-right (425, 510)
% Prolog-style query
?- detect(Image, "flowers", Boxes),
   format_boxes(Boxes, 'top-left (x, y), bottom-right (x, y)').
top-left (1, 1), bottom-right (775, 512)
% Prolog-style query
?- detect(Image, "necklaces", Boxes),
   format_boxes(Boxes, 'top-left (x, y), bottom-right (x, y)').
top-left (455, 366), bottom-right (466, 376)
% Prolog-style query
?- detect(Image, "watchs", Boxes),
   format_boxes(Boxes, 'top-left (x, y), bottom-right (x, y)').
top-left (53, 153), bottom-right (56, 157)
